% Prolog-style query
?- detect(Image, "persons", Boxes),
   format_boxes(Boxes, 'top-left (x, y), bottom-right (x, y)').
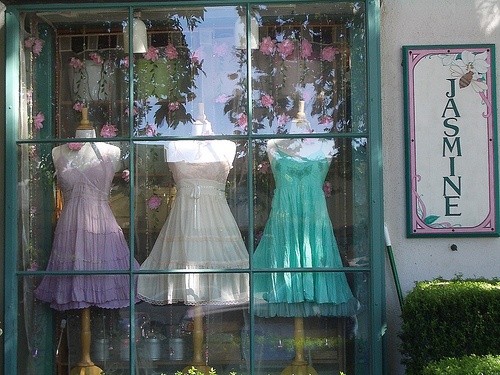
top-left (250, 122), bottom-right (355, 318)
top-left (33, 130), bottom-right (141, 310)
top-left (138, 125), bottom-right (250, 305)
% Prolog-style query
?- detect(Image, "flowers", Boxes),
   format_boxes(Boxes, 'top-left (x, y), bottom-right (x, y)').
top-left (24, 37), bottom-right (339, 280)
